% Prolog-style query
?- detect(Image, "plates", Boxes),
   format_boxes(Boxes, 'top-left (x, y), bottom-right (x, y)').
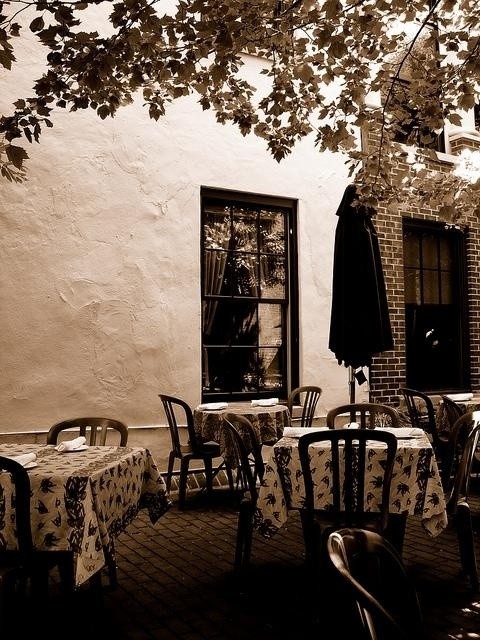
top-left (54, 444), bottom-right (89, 452)
top-left (22, 461), bottom-right (38, 470)
top-left (258, 403), bottom-right (276, 407)
top-left (205, 407), bottom-right (223, 411)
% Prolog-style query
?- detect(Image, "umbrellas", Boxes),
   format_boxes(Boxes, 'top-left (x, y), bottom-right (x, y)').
top-left (329, 182), bottom-right (393, 425)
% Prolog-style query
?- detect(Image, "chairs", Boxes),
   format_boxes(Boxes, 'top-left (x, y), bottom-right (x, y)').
top-left (0, 456), bottom-right (77, 625)
top-left (47, 418), bottom-right (129, 447)
top-left (253, 385), bottom-right (323, 490)
top-left (157, 393), bottom-right (236, 510)
top-left (299, 386), bottom-right (480, 640)
